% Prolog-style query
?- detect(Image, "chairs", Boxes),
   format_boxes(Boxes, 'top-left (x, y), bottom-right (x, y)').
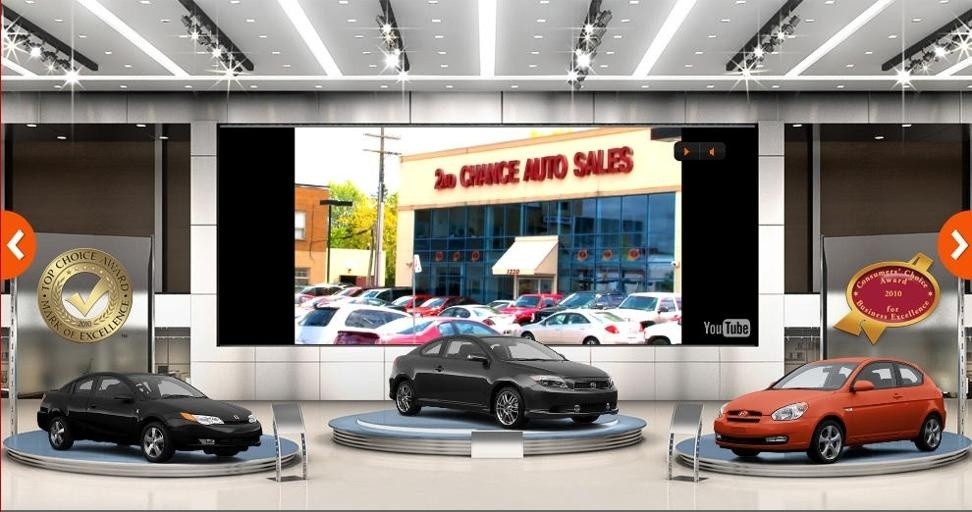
top-left (104, 382), bottom-right (134, 396)
top-left (825, 371), bottom-right (911, 388)
top-left (453, 343), bottom-right (510, 360)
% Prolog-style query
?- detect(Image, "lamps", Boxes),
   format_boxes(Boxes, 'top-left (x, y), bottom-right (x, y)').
top-left (180, 12), bottom-right (244, 77)
top-left (904, 25), bottom-right (972, 84)
top-left (569, 10), bottom-right (614, 90)
top-left (4, 23), bottom-right (79, 85)
top-left (376, 13), bottom-right (408, 45)
top-left (738, 15), bottom-right (801, 75)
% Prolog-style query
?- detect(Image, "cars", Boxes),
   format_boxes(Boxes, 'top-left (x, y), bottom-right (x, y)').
top-left (37, 372), bottom-right (263, 463)
top-left (389, 334), bottom-right (619, 428)
top-left (714, 357), bottom-right (947, 463)
